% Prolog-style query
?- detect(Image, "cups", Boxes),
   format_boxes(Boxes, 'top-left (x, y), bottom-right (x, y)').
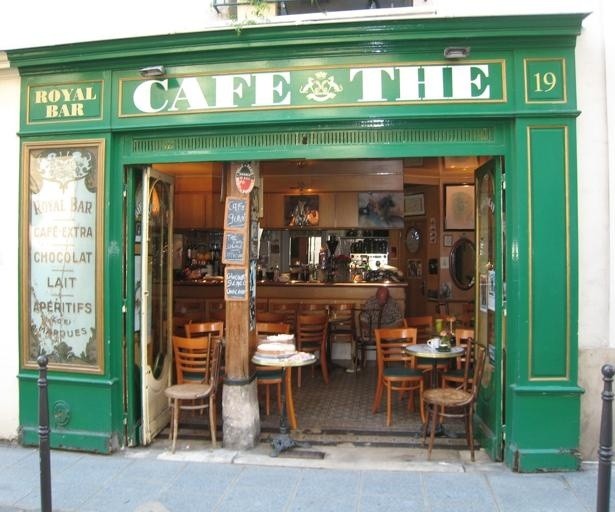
top-left (426, 337), bottom-right (441, 350)
top-left (435, 318), bottom-right (444, 333)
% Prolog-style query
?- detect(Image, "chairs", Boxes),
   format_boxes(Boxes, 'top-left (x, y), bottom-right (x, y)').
top-left (373, 297), bottom-right (489, 426)
top-left (162, 296), bottom-right (330, 418)
top-left (349, 307), bottom-right (385, 371)
top-left (163, 337), bottom-right (223, 454)
top-left (421, 348), bottom-right (488, 463)
top-left (329, 302), bottom-right (355, 364)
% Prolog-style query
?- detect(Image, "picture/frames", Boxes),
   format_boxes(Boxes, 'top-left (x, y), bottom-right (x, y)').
top-left (403, 190), bottom-right (427, 219)
top-left (442, 182), bottom-right (475, 233)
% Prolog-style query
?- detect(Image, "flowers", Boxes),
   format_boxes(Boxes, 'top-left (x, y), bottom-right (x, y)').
top-left (335, 254), bottom-right (352, 273)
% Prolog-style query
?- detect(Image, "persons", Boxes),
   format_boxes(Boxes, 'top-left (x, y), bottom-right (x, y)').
top-left (346, 285), bottom-right (404, 374)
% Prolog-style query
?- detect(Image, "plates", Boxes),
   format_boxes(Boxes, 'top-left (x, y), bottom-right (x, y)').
top-left (257, 342), bottom-right (297, 362)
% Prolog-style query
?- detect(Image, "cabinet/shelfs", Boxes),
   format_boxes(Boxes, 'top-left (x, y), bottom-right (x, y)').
top-left (250, 347), bottom-right (317, 432)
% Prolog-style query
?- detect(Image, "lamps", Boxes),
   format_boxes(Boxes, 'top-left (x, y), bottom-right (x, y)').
top-left (443, 43), bottom-right (473, 61)
top-left (138, 63), bottom-right (168, 78)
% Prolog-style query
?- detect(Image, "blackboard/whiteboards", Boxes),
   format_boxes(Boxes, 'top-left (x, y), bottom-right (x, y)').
top-left (221, 196), bottom-right (249, 302)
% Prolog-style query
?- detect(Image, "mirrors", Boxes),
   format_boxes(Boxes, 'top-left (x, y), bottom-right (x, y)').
top-left (449, 237), bottom-right (476, 291)
